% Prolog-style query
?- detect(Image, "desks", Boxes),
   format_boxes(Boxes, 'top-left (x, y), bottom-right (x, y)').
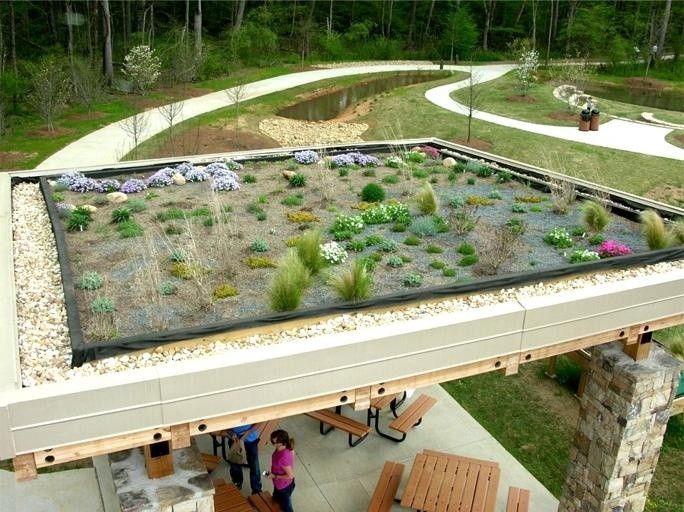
top-left (336, 391), bottom-right (407, 433)
top-left (399, 455), bottom-right (502, 512)
top-left (213, 483), bottom-right (254, 512)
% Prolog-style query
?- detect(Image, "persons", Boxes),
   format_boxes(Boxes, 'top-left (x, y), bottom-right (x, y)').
top-left (225, 423), bottom-right (262, 495)
top-left (267, 429), bottom-right (295, 512)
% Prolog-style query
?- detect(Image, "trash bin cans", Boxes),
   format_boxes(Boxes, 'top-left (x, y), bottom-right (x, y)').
top-left (579, 109), bottom-right (600, 131)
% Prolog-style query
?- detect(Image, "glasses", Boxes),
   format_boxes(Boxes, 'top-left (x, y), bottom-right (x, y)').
top-left (271, 440), bottom-right (283, 444)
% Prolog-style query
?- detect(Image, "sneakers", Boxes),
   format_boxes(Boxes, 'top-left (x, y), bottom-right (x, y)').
top-left (236, 484), bottom-right (242, 490)
top-left (251, 490), bottom-right (262, 495)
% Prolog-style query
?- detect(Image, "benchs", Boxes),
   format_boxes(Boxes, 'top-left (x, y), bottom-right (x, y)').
top-left (366, 460), bottom-right (406, 512)
top-left (193, 453), bottom-right (223, 481)
top-left (305, 409), bottom-right (373, 447)
top-left (388, 393), bottom-right (438, 443)
top-left (505, 486), bottom-right (530, 511)
top-left (247, 491), bottom-right (283, 512)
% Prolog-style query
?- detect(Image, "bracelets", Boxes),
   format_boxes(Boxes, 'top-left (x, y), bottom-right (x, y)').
top-left (275, 475), bottom-right (277, 479)
